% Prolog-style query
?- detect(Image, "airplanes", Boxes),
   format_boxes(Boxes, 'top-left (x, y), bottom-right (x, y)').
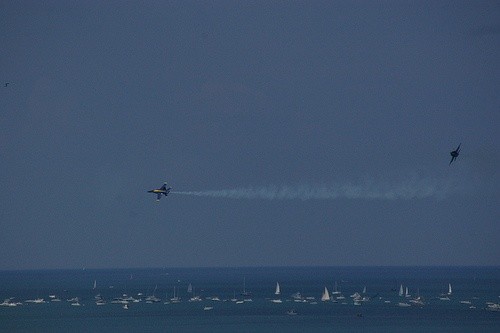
top-left (147, 183), bottom-right (172, 201)
top-left (449, 142), bottom-right (463, 167)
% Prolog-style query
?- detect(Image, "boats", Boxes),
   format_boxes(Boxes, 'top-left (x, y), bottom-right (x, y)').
top-left (1, 277), bottom-right (500, 317)
